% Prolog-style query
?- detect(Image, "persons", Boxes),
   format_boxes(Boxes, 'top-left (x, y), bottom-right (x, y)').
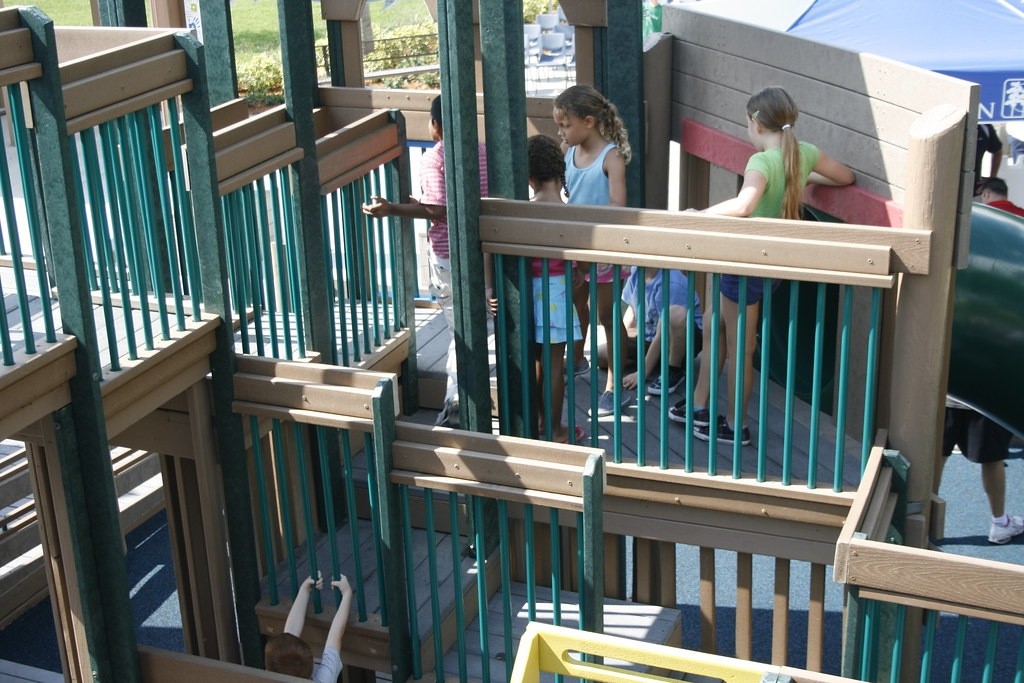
top-left (671, 83), bottom-right (857, 445)
top-left (481, 133), bottom-right (585, 446)
top-left (261, 565), bottom-right (353, 683)
top-left (978, 177), bottom-right (1023, 227)
top-left (592, 261), bottom-right (706, 395)
top-left (360, 93), bottom-right (497, 430)
top-left (546, 84), bottom-right (634, 419)
top-left (936, 397), bottom-right (1023, 545)
top-left (972, 122), bottom-right (1003, 196)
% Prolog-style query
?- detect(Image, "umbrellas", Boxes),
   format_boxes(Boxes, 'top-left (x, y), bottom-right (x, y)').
top-left (660, 0), bottom-right (1024, 83)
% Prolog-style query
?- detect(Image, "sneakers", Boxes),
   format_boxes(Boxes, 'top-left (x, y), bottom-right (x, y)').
top-left (668, 399), bottom-right (709, 426)
top-left (693, 415), bottom-right (751, 446)
top-left (647, 364), bottom-right (685, 395)
top-left (588, 390), bottom-right (631, 417)
top-left (562, 357), bottom-right (589, 376)
top-left (988, 515), bottom-right (1024, 544)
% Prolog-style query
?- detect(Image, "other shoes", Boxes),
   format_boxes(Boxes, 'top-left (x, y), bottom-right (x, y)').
top-left (564, 425), bottom-right (585, 444)
top-left (435, 397), bottom-right (460, 429)
